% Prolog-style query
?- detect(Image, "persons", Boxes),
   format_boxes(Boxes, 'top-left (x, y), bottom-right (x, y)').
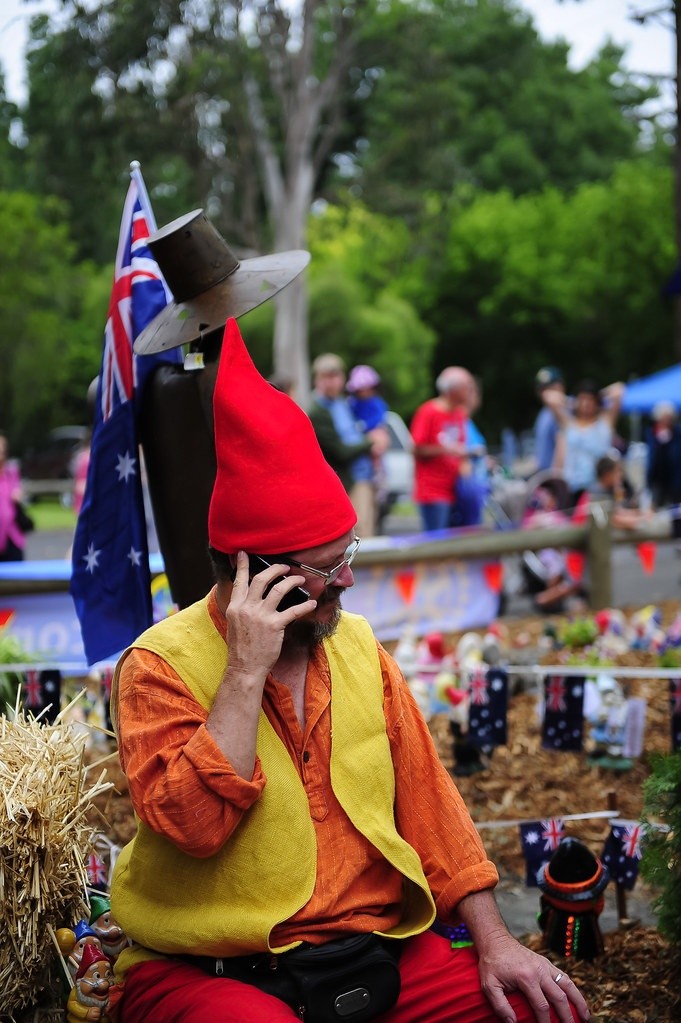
top-left (303, 352), bottom-right (497, 541)
top-left (68, 921), bottom-right (102, 984)
top-left (110, 315), bottom-right (590, 1023)
top-left (90, 895), bottom-right (129, 967)
top-left (67, 942), bottom-right (117, 1023)
top-left (76, 375), bottom-right (100, 513)
top-left (0, 436), bottom-right (27, 560)
top-left (504, 363), bottom-right (681, 617)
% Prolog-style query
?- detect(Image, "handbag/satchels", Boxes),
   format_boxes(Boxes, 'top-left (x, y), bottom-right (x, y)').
top-left (14, 501), bottom-right (33, 531)
top-left (137, 933), bottom-right (409, 1023)
top-left (208, 316), bottom-right (357, 555)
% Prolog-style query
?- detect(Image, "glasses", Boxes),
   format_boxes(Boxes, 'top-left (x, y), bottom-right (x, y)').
top-left (265, 535), bottom-right (361, 586)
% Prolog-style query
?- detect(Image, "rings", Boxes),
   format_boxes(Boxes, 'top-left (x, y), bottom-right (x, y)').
top-left (555, 973), bottom-right (563, 983)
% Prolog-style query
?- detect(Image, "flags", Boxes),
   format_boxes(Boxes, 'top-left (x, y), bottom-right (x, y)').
top-left (69, 180), bottom-right (187, 668)
top-left (543, 674), bottom-right (584, 752)
top-left (518, 819), bottom-right (569, 887)
top-left (466, 668), bottom-right (507, 746)
top-left (601, 819), bottom-right (648, 893)
top-left (86, 856), bottom-right (107, 885)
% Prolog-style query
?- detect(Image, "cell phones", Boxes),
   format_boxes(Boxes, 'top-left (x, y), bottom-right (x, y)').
top-left (227, 554), bottom-right (310, 628)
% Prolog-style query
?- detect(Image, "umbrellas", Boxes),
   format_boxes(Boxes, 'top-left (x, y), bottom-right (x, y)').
top-left (617, 369), bottom-right (681, 415)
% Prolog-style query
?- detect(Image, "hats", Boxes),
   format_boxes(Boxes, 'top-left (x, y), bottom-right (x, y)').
top-left (314, 353), bottom-right (345, 374)
top-left (534, 367), bottom-right (566, 393)
top-left (346, 366), bottom-right (380, 392)
